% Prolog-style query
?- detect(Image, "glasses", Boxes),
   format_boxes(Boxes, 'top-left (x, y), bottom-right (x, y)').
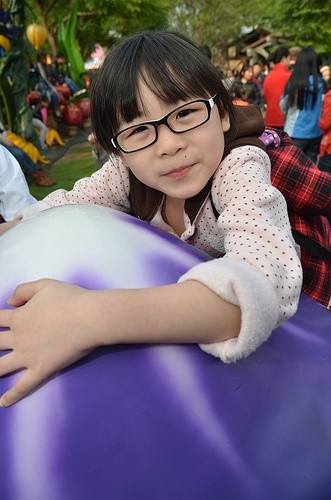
top-left (112, 96), bottom-right (216, 157)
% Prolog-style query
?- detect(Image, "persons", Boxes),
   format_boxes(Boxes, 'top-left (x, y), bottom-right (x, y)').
top-left (198, 45), bottom-right (331, 174)
top-left (0, 31), bottom-right (303, 409)
top-left (0, 43), bottom-right (106, 223)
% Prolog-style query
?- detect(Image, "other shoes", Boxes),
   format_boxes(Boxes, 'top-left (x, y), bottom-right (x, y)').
top-left (38, 169), bottom-right (56, 183)
top-left (32, 171), bottom-right (53, 187)
